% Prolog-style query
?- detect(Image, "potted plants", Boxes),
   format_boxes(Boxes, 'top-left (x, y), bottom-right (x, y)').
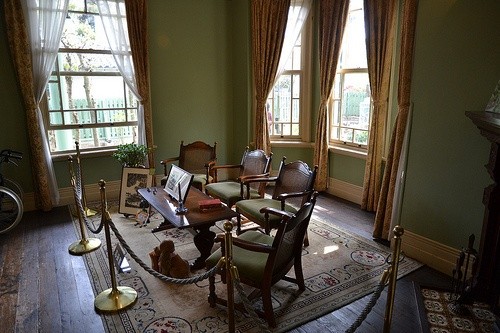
top-left (112, 143), bottom-right (155, 169)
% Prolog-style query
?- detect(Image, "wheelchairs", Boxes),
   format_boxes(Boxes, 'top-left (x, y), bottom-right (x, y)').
top-left (0, 148), bottom-right (24, 235)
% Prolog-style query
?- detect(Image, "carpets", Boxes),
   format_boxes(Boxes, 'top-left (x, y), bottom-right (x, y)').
top-left (68, 193), bottom-right (425, 333)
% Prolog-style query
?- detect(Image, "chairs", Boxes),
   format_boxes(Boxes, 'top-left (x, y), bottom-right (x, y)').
top-left (236, 156), bottom-right (320, 247)
top-left (205, 191), bottom-right (319, 328)
top-left (160, 140), bottom-right (218, 193)
top-left (204, 146), bottom-right (274, 222)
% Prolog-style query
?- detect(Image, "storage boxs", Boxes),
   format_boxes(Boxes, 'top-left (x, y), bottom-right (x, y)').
top-left (197, 199), bottom-right (222, 209)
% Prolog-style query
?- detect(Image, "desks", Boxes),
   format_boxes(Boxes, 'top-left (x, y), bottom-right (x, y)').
top-left (465, 110), bottom-right (500, 319)
top-left (138, 184), bottom-right (239, 271)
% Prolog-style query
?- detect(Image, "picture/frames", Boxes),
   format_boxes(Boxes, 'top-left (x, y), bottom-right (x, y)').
top-left (163, 163), bottom-right (194, 204)
top-left (118, 167), bottom-right (153, 215)
